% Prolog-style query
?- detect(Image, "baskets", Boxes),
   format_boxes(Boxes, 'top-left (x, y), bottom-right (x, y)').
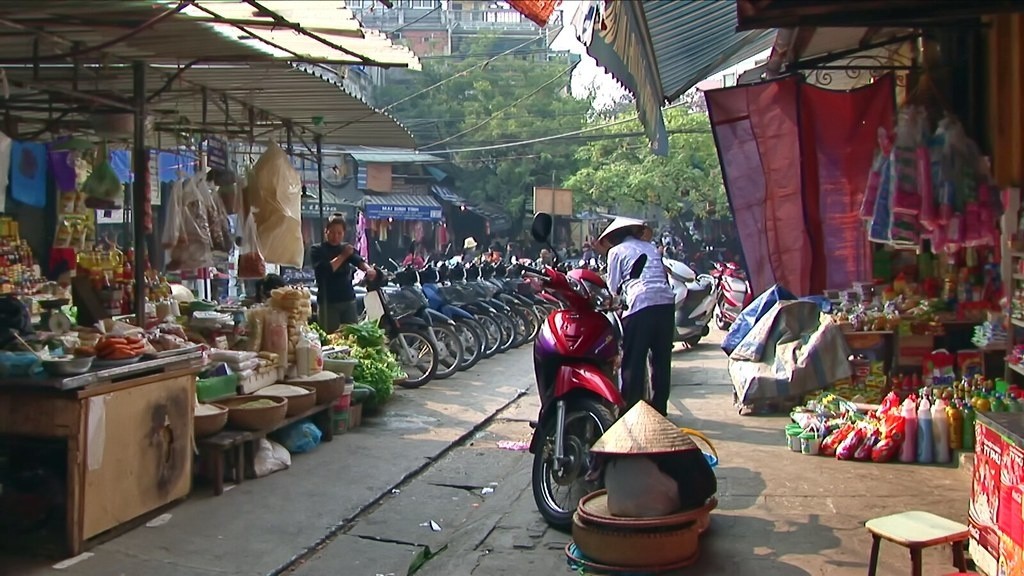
top-left (194, 370), bottom-right (345, 438)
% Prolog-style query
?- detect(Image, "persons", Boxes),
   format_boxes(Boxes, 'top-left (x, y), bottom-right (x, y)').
top-left (596, 212), bottom-right (675, 421)
top-left (309, 213), bottom-right (377, 336)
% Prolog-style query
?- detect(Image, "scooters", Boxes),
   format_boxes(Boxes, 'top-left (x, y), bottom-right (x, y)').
top-left (661, 256), bottom-right (754, 350)
top-left (514, 209), bottom-right (629, 534)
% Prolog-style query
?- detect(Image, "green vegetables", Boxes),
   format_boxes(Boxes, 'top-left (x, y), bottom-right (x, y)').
top-left (307, 317), bottom-right (405, 411)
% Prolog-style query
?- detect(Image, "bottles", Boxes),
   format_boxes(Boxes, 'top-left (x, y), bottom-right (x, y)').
top-left (0, 212), bottom-right (53, 299)
top-left (881, 373), bottom-right (1024, 462)
top-left (872, 247), bottom-right (969, 304)
top-left (76, 243), bottom-right (172, 319)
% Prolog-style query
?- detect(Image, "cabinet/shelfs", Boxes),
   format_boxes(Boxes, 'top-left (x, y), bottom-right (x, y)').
top-left (1001, 188), bottom-right (1024, 393)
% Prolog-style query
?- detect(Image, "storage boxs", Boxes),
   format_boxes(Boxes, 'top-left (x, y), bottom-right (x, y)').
top-left (895, 333), bottom-right (935, 367)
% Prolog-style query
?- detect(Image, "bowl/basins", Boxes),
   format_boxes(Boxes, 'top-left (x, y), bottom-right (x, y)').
top-left (42, 355), bottom-right (95, 374)
top-left (2, 353), bottom-right (43, 375)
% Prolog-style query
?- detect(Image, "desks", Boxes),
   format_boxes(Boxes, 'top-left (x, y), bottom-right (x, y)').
top-left (969, 410), bottom-right (1024, 576)
top-left (0, 344), bottom-right (200, 556)
top-left (194, 400), bottom-right (339, 497)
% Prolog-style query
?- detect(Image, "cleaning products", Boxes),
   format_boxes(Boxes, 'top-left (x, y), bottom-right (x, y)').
top-left (785, 349), bottom-right (1024, 463)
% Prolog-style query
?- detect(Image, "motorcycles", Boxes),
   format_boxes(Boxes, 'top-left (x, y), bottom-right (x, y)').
top-left (282, 240), bottom-right (607, 391)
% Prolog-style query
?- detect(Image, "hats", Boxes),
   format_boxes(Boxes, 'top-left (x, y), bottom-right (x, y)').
top-left (590, 399), bottom-right (698, 453)
top-left (690, 261), bottom-right (696, 265)
top-left (594, 217), bottom-right (653, 257)
top-left (583, 243), bottom-right (591, 248)
top-left (540, 249), bottom-right (551, 258)
top-left (464, 237), bottom-right (477, 248)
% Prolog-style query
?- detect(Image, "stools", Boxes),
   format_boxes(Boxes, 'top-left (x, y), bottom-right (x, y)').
top-left (864, 510), bottom-right (970, 576)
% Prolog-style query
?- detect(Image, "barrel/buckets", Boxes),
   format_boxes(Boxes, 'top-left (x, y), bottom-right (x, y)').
top-left (330, 411), bottom-right (349, 434)
top-left (333, 393), bottom-right (351, 411)
top-left (785, 423), bottom-right (821, 456)
